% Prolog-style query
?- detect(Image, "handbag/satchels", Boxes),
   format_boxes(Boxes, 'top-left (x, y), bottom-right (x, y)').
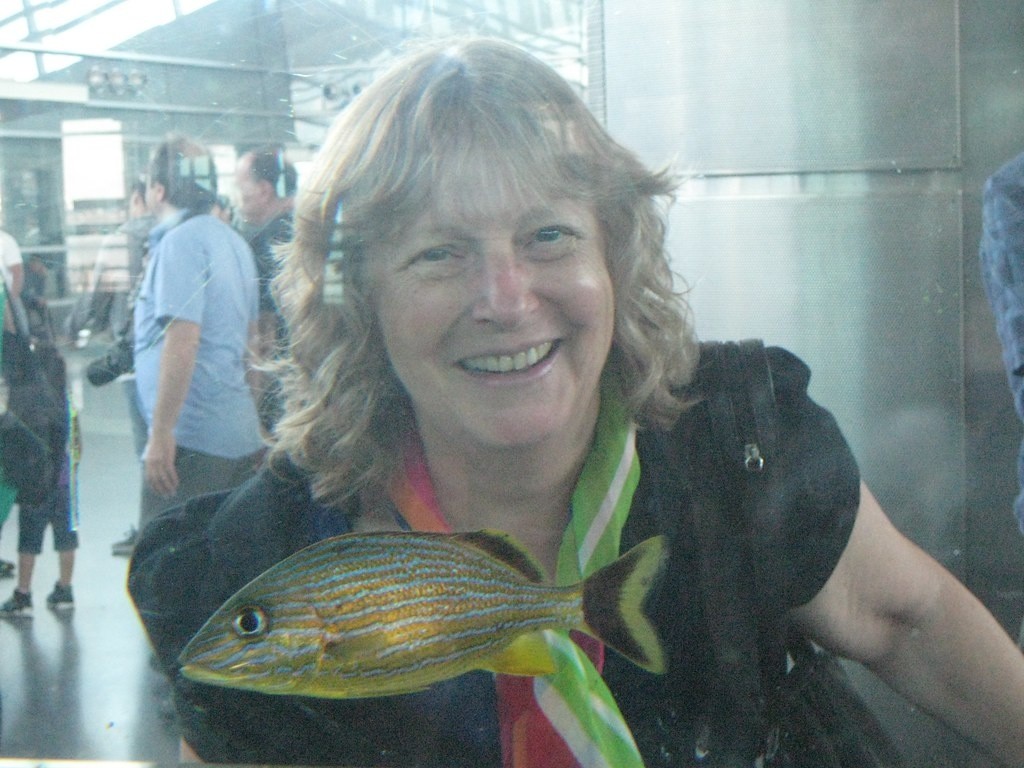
top-left (656, 338), bottom-right (910, 768)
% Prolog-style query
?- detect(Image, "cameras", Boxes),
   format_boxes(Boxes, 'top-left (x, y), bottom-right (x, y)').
top-left (86, 315), bottom-right (138, 386)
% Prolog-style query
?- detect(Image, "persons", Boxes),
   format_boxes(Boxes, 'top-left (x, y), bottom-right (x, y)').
top-left (0, 202), bottom-right (78, 617)
top-left (118, 140), bottom-right (298, 558)
top-left (978, 154), bottom-right (1023, 423)
top-left (128, 36), bottom-right (1024, 768)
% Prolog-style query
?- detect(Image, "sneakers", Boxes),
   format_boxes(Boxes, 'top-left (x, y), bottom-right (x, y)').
top-left (48, 581), bottom-right (72, 611)
top-left (0, 589), bottom-right (34, 618)
top-left (111, 526), bottom-right (138, 554)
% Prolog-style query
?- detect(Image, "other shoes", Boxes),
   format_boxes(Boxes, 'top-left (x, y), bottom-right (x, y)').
top-left (0, 559), bottom-right (14, 573)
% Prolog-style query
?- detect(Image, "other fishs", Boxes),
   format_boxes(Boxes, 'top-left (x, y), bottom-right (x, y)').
top-left (174, 527), bottom-right (676, 698)
